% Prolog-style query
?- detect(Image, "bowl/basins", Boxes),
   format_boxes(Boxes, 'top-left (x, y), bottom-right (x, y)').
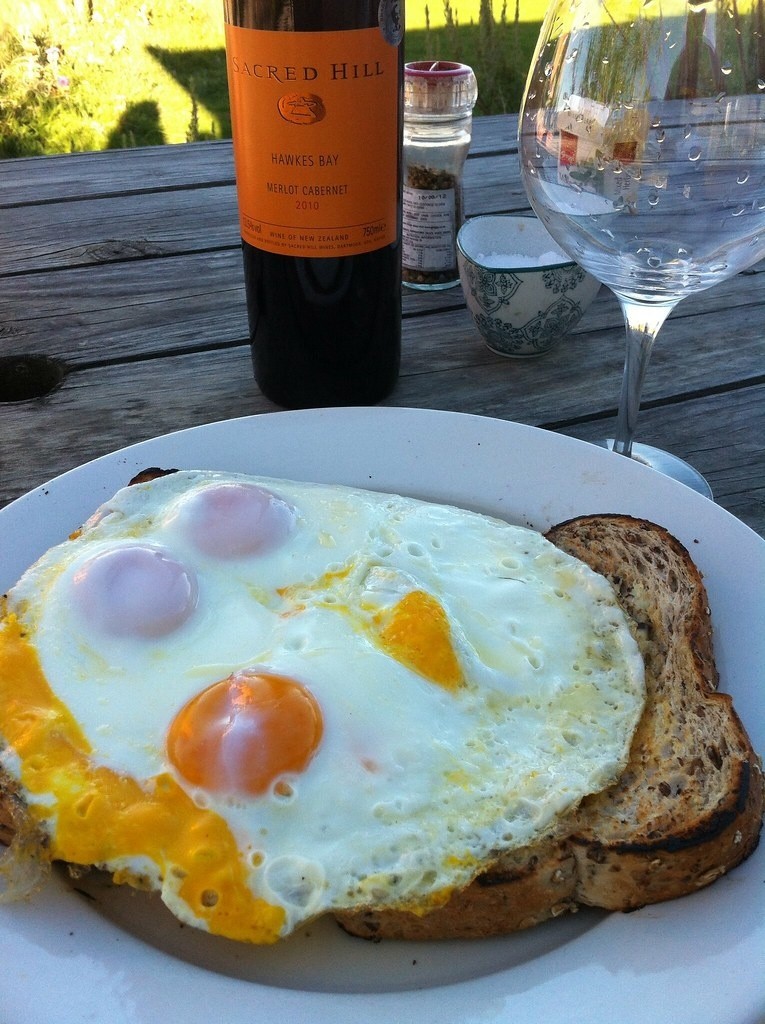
top-left (455, 214), bottom-right (602, 358)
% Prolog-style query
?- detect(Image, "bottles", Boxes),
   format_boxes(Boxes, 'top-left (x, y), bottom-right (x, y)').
top-left (224, 0), bottom-right (406, 406)
top-left (403, 61), bottom-right (478, 292)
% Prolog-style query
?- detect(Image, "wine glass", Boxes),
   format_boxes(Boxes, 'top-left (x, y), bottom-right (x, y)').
top-left (514, 0), bottom-right (765, 505)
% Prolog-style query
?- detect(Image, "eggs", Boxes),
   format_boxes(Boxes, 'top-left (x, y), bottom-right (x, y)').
top-left (0, 470), bottom-right (649, 943)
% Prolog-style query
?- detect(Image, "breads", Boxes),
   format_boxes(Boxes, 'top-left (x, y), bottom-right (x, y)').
top-left (0, 465), bottom-right (576, 942)
top-left (532, 513), bottom-right (764, 909)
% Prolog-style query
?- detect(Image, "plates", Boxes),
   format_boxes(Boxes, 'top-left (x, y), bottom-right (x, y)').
top-left (0, 408), bottom-right (765, 1024)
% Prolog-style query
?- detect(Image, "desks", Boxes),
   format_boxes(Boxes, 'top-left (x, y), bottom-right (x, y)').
top-left (0, 99), bottom-right (765, 1024)
